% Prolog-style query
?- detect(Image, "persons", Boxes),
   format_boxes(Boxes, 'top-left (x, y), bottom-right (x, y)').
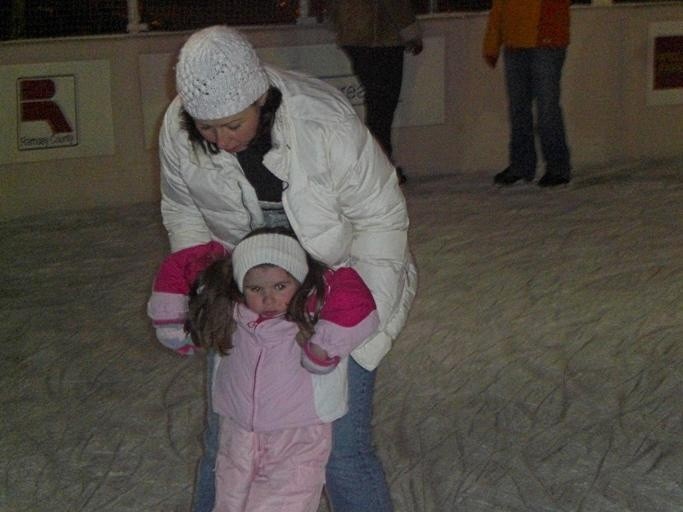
top-left (146, 224), bottom-right (380, 511)
top-left (482, 0), bottom-right (573, 187)
top-left (333, 0), bottom-right (424, 187)
top-left (155, 22), bottom-right (422, 512)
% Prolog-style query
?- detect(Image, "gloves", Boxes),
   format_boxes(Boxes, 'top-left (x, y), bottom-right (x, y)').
top-left (405, 38), bottom-right (421, 55)
top-left (310, 267), bottom-right (380, 358)
top-left (146, 240), bottom-right (231, 325)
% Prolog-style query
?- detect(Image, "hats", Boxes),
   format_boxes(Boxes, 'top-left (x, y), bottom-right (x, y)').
top-left (233, 225), bottom-right (310, 293)
top-left (174, 22), bottom-right (271, 120)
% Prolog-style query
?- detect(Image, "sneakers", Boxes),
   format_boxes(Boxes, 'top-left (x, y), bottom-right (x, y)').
top-left (494, 162), bottom-right (536, 186)
top-left (536, 160), bottom-right (571, 188)
